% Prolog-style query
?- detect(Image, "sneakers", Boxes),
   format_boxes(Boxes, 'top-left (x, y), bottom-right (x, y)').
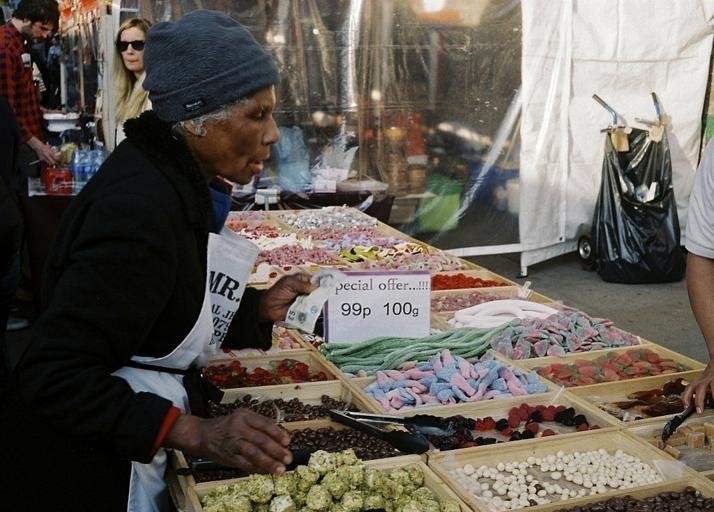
top-left (5, 300), bottom-right (29, 331)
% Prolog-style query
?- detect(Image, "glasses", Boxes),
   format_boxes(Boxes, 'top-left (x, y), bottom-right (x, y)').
top-left (116, 41), bottom-right (145, 53)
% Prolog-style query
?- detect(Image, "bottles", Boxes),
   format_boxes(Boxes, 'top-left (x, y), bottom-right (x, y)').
top-left (73, 140), bottom-right (107, 196)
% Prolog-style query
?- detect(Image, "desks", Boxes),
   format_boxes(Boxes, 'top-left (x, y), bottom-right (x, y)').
top-left (25, 176), bottom-right (94, 317)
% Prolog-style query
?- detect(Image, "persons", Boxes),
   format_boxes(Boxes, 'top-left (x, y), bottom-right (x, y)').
top-left (677, 135), bottom-right (712, 413)
top-left (0, 0), bottom-right (62, 329)
top-left (0, 13), bottom-right (319, 510)
top-left (111, 19), bottom-right (153, 151)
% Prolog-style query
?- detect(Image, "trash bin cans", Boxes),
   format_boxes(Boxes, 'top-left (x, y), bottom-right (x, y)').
top-left (418, 173), bottom-right (464, 233)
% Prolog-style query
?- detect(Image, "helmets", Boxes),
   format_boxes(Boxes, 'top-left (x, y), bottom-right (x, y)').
top-left (141, 8), bottom-right (279, 124)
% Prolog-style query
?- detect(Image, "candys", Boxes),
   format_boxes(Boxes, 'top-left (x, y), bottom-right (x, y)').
top-left (182, 206), bottom-right (714, 512)
